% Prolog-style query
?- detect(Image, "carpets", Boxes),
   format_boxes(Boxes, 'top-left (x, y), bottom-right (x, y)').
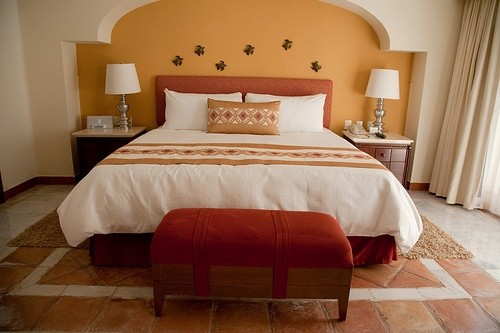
top-left (8, 204), bottom-right (91, 249)
top-left (397, 213), bottom-right (476, 260)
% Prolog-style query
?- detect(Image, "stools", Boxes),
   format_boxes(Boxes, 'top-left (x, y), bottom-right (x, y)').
top-left (149, 208), bottom-right (353, 322)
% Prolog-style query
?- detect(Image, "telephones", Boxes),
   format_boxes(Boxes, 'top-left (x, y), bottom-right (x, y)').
top-left (349, 124), bottom-right (370, 138)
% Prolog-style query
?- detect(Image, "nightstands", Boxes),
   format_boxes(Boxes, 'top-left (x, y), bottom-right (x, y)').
top-left (342, 130), bottom-right (414, 190)
top-left (71, 126), bottom-right (148, 187)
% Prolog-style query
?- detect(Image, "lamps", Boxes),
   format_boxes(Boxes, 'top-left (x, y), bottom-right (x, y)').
top-left (364, 69), bottom-right (400, 135)
top-left (105, 63), bottom-right (142, 130)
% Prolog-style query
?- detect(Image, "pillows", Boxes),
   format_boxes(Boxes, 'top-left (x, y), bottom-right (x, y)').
top-left (207, 97), bottom-right (281, 135)
top-left (245, 93), bottom-right (327, 133)
top-left (163, 88), bottom-right (243, 131)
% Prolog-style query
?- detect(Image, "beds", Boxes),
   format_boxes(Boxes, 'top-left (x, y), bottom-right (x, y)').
top-left (57, 75), bottom-right (423, 267)
